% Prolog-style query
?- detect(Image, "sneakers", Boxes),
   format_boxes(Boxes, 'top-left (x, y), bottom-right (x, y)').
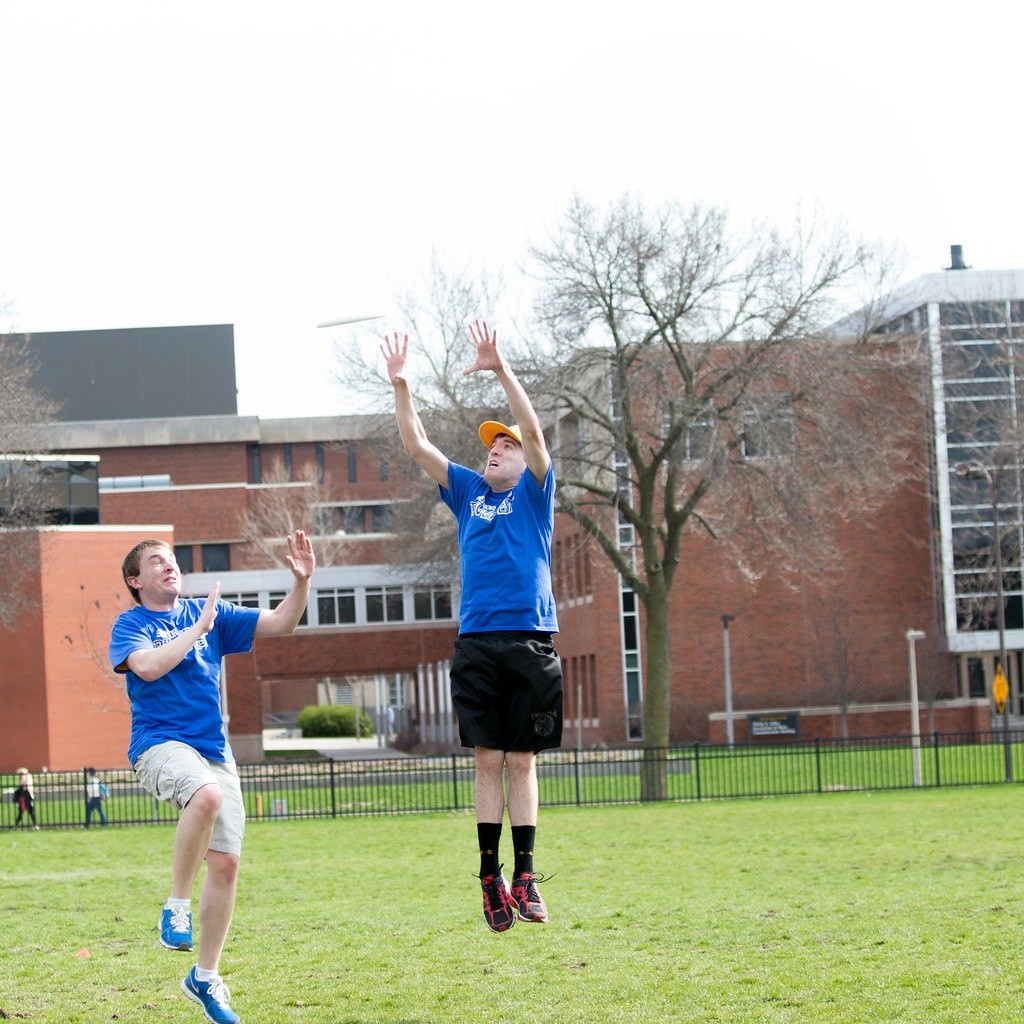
top-left (480, 871), bottom-right (517, 936)
top-left (179, 963), bottom-right (239, 1024)
top-left (506, 871), bottom-right (549, 924)
top-left (155, 902), bottom-right (195, 952)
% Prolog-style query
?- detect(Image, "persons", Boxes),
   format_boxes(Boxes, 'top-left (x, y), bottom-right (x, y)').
top-left (11, 767), bottom-right (41, 832)
top-left (379, 319), bottom-right (563, 934)
top-left (80, 767), bottom-right (108, 830)
top-left (108, 528), bottom-right (316, 1024)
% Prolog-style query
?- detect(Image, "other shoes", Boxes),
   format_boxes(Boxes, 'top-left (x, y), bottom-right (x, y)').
top-left (80, 825), bottom-right (91, 831)
top-left (13, 827), bottom-right (21, 832)
top-left (97, 824), bottom-right (107, 829)
top-left (32, 825), bottom-right (40, 831)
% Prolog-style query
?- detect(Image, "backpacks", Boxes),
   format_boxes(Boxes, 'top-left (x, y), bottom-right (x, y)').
top-left (98, 783), bottom-right (110, 802)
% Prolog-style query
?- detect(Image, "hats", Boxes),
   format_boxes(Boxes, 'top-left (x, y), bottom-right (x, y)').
top-left (478, 420), bottom-right (524, 448)
top-left (16, 766), bottom-right (29, 775)
